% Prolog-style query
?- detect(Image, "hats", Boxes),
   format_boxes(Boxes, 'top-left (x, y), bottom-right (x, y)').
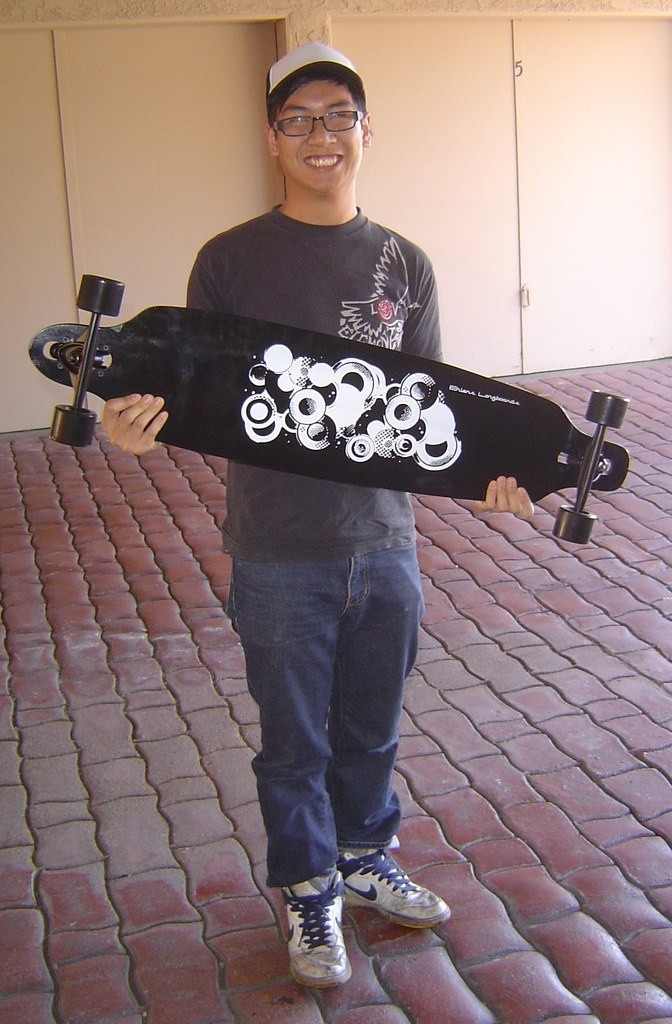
top-left (265, 40), bottom-right (366, 98)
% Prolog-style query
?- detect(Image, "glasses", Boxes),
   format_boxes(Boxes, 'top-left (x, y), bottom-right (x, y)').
top-left (271, 110), bottom-right (362, 138)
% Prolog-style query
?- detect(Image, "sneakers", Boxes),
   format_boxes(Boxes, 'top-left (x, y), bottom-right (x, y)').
top-left (336, 846), bottom-right (453, 930)
top-left (281, 866), bottom-right (354, 989)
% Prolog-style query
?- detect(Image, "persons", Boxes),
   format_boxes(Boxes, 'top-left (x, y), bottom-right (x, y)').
top-left (103, 43), bottom-right (540, 990)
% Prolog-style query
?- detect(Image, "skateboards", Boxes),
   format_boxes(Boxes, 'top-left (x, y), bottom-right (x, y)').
top-left (27, 272), bottom-right (630, 546)
